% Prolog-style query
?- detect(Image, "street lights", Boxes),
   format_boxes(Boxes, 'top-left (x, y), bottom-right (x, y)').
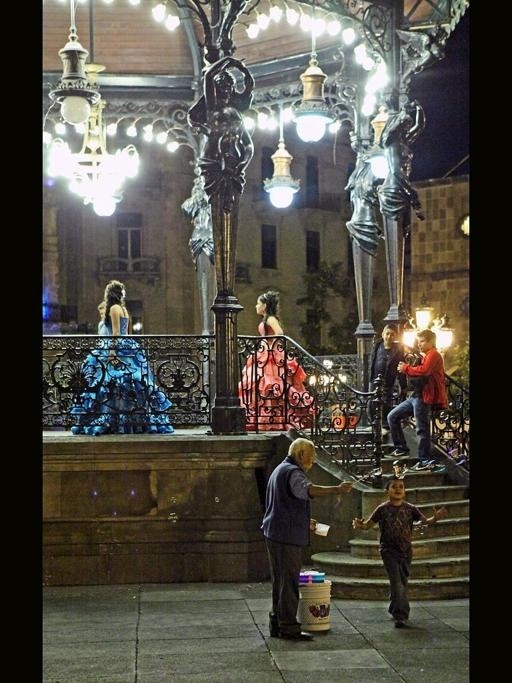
top-left (402, 292), bottom-right (456, 371)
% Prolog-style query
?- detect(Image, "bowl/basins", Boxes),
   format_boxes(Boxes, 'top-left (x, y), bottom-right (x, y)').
top-left (314, 523), bottom-right (330, 536)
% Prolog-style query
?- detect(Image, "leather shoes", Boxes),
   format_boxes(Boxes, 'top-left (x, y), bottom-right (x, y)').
top-left (268, 629), bottom-right (313, 640)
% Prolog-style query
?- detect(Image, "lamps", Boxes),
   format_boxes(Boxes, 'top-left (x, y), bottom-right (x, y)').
top-left (143, 0), bottom-right (391, 153)
top-left (42, 0), bottom-right (140, 216)
top-left (262, 111), bottom-right (300, 209)
top-left (46, 0), bottom-right (101, 125)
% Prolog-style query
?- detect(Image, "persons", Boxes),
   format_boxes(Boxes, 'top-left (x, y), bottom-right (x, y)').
top-left (259, 435), bottom-right (351, 642)
top-left (379, 99), bottom-right (425, 222)
top-left (352, 475), bottom-right (447, 623)
top-left (366, 326), bottom-right (404, 442)
top-left (199, 54), bottom-right (255, 213)
top-left (72, 279), bottom-right (176, 435)
top-left (386, 330), bottom-right (447, 469)
top-left (238, 291), bottom-right (312, 429)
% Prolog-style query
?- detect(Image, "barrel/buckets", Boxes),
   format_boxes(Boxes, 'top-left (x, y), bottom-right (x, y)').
top-left (297, 581), bottom-right (332, 632)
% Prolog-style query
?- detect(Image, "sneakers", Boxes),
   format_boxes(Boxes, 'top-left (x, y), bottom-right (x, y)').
top-left (408, 462), bottom-right (431, 473)
top-left (384, 448), bottom-right (409, 459)
top-left (394, 618), bottom-right (409, 628)
